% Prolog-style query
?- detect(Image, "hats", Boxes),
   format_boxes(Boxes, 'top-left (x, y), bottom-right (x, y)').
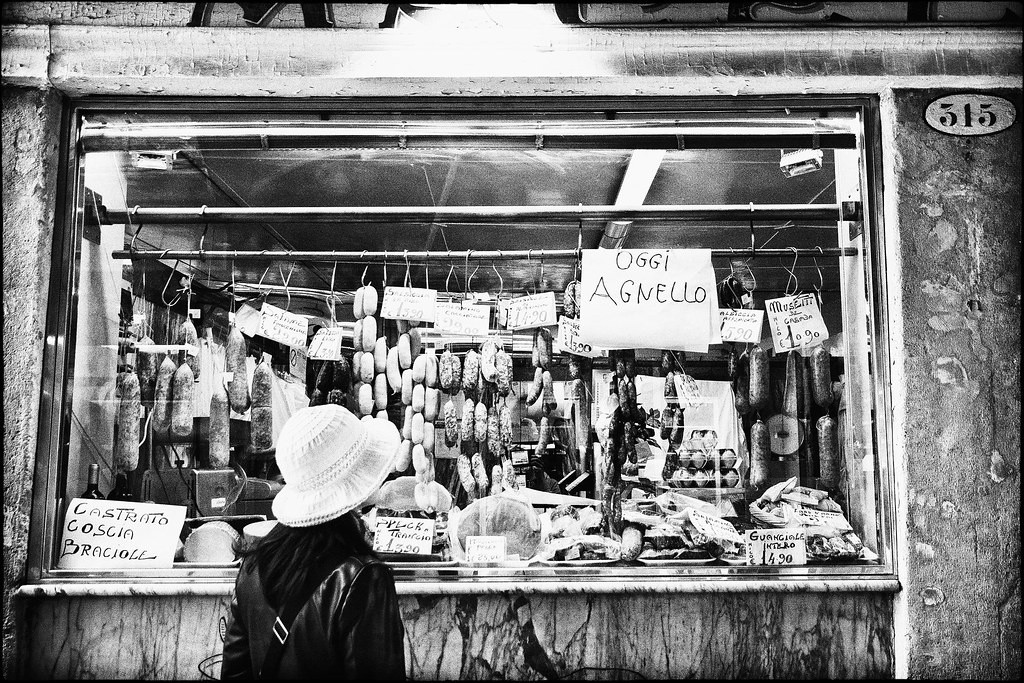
top-left (271, 403), bottom-right (402, 528)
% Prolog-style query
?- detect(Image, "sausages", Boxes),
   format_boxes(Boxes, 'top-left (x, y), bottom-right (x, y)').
top-left (116, 285), bottom-right (843, 565)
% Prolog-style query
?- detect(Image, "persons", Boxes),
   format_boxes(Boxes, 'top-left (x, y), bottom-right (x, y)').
top-left (216, 404), bottom-right (415, 683)
